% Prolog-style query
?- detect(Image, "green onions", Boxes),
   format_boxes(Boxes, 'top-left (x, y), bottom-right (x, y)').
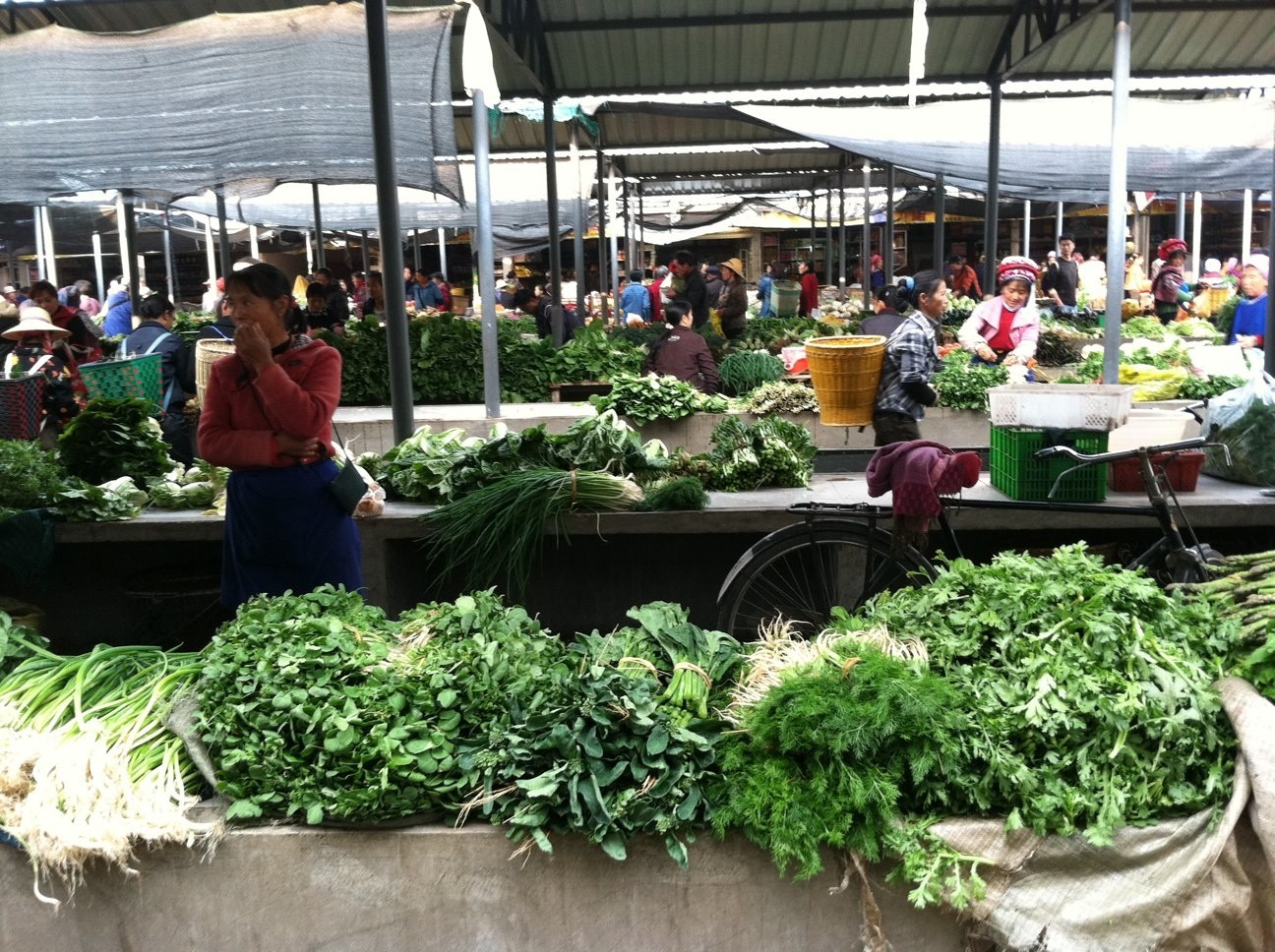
top-left (410, 470), bottom-right (647, 604)
top-left (1, 630), bottom-right (209, 894)
top-left (715, 348), bottom-right (785, 394)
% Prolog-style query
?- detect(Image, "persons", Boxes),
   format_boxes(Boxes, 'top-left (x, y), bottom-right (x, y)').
top-left (115, 294), bottom-right (190, 464)
top-left (619, 250), bottom-right (748, 349)
top-left (796, 260), bottom-right (818, 318)
top-left (195, 262), bottom-right (366, 618)
top-left (756, 265), bottom-right (786, 318)
top-left (644, 300), bottom-right (719, 395)
top-left (0, 306), bottom-right (78, 451)
top-left (943, 253), bottom-right (1039, 382)
top-left (1036, 228), bottom-right (1147, 313)
top-left (0, 275), bottom-right (225, 337)
top-left (185, 292), bottom-right (236, 462)
top-left (495, 254), bottom-right (610, 345)
top-left (1151, 238), bottom-right (1269, 326)
top-left (292, 265), bottom-right (451, 338)
top-left (1228, 254), bottom-right (1269, 351)
top-left (855, 269), bottom-right (950, 446)
top-left (870, 254), bottom-right (884, 289)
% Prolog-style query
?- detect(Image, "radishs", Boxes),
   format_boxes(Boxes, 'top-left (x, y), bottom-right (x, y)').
top-left (818, 298), bottom-right (864, 318)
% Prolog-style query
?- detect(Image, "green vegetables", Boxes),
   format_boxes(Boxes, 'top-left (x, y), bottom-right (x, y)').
top-left (1040, 313), bottom-right (1275, 487)
top-left (733, 317), bottom-right (864, 352)
top-left (0, 395), bottom-right (233, 523)
top-left (169, 309), bottom-right (216, 347)
top-left (349, 409), bottom-right (817, 511)
top-left (925, 348), bottom-right (1010, 412)
top-left (326, 313), bottom-right (730, 428)
top-left (198, 537), bottom-right (1240, 915)
top-left (735, 379), bottom-right (821, 414)
top-left (0, 591), bottom-right (55, 676)
top-left (1239, 617), bottom-right (1275, 707)
top-left (938, 295), bottom-right (976, 328)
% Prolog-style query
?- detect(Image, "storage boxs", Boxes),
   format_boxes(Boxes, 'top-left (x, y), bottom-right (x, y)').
top-left (985, 380), bottom-right (1207, 502)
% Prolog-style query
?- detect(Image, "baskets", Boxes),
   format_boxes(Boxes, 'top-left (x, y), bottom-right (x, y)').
top-left (1200, 286), bottom-right (1232, 311)
top-left (195, 336), bottom-right (238, 412)
top-left (987, 382), bottom-right (1135, 429)
top-left (79, 333), bottom-right (172, 421)
top-left (771, 278), bottom-right (802, 316)
top-left (0, 349), bottom-right (52, 443)
top-left (806, 337), bottom-right (885, 425)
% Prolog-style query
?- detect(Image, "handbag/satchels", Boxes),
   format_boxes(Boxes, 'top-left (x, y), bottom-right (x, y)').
top-left (325, 460), bottom-right (369, 514)
top-left (334, 443), bottom-right (386, 517)
top-left (1196, 352), bottom-right (1275, 486)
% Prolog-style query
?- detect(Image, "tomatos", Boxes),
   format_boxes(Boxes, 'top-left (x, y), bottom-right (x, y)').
top-left (937, 342), bottom-right (961, 358)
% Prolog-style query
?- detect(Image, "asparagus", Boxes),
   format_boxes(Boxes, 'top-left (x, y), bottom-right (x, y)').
top-left (1162, 549), bottom-right (1275, 645)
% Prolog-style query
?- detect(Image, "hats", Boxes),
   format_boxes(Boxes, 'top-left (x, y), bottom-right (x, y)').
top-left (56, 285), bottom-right (81, 307)
top-left (3, 286), bottom-right (15, 294)
top-left (1155, 240), bottom-right (1189, 261)
top-left (2, 306), bottom-right (70, 341)
top-left (1243, 254), bottom-right (1270, 279)
top-left (217, 279), bottom-right (225, 289)
top-left (721, 259), bottom-right (749, 282)
top-left (996, 256), bottom-right (1042, 283)
top-left (203, 278), bottom-right (210, 284)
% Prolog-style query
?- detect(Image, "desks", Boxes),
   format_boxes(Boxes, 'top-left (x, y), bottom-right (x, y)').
top-left (550, 374), bottom-right (811, 403)
top-left (51, 468), bottom-right (1275, 622)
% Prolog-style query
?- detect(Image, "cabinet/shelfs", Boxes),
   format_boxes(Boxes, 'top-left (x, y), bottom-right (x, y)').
top-left (845, 226), bottom-right (908, 284)
top-left (1201, 215), bottom-right (1267, 251)
top-left (973, 235), bottom-right (1106, 259)
top-left (147, 260), bottom-right (205, 292)
top-left (739, 246), bottom-right (826, 278)
